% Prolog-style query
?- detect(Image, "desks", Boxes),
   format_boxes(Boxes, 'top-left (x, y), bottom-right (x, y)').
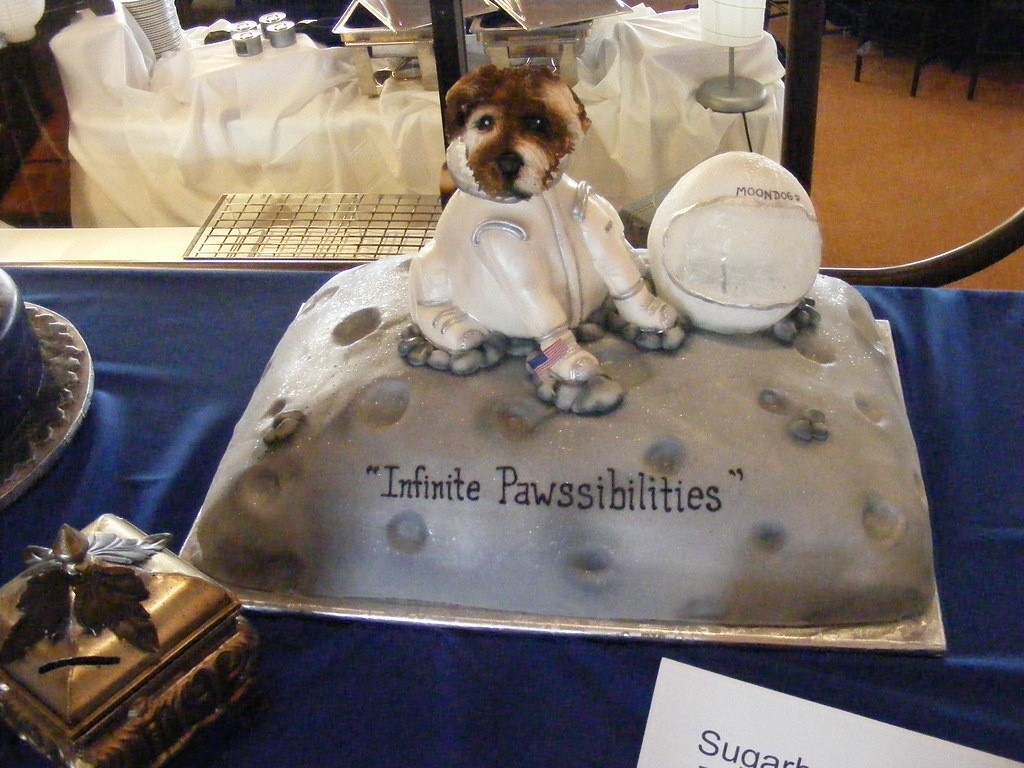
top-left (0, 267), bottom-right (1024, 766)
top-left (69, 20), bottom-right (787, 229)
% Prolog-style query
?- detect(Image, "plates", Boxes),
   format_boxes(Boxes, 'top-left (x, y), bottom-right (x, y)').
top-left (119, 0), bottom-right (182, 62)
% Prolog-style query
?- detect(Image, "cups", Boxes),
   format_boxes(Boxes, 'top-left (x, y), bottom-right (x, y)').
top-left (1, 272), bottom-right (42, 462)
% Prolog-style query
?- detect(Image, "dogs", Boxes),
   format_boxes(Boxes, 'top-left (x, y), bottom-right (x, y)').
top-left (444, 61), bottom-right (595, 202)
